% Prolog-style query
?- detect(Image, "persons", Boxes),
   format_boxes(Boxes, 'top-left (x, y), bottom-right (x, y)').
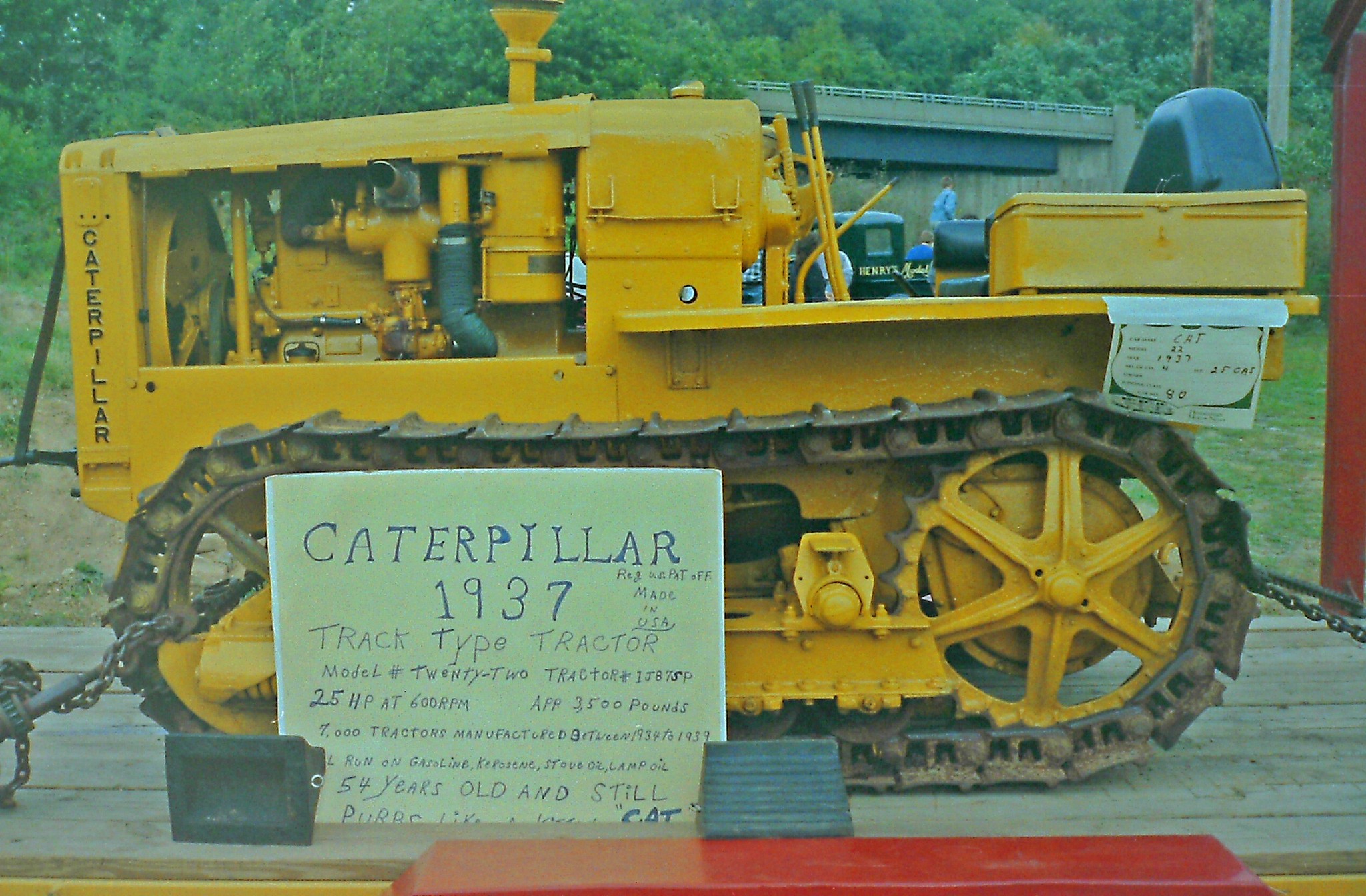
top-left (742, 247), bottom-right (764, 306)
top-left (906, 230), bottom-right (934, 267)
top-left (928, 176), bottom-right (957, 284)
top-left (789, 228), bottom-right (853, 303)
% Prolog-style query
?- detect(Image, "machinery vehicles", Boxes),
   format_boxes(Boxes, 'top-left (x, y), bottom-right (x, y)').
top-left (0, 2), bottom-right (1364, 817)
